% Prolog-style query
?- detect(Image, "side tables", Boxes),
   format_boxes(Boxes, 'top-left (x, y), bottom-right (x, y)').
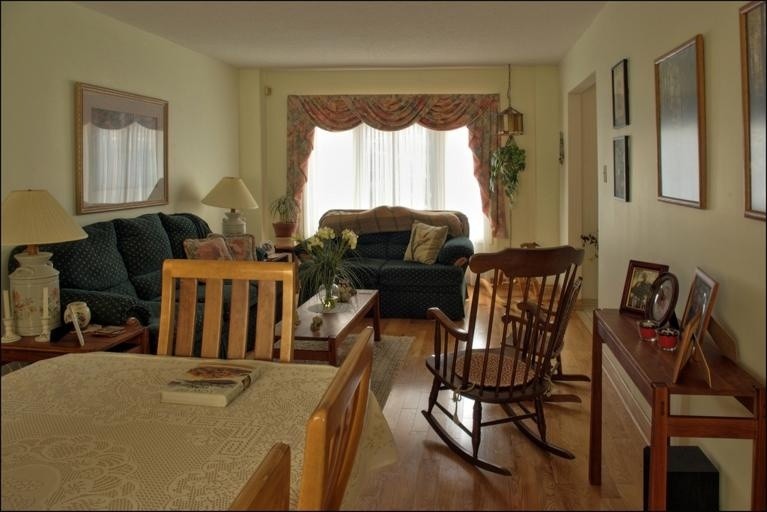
top-left (0, 324), bottom-right (149, 375)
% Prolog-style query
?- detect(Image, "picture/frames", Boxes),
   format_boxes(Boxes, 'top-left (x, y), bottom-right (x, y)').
top-left (672, 315), bottom-right (712, 389)
top-left (652, 33), bottom-right (707, 211)
top-left (611, 57), bottom-right (631, 130)
top-left (611, 136), bottom-right (631, 204)
top-left (737, 1), bottom-right (766, 223)
top-left (74, 79), bottom-right (170, 215)
top-left (619, 258), bottom-right (670, 322)
top-left (680, 266), bottom-right (718, 346)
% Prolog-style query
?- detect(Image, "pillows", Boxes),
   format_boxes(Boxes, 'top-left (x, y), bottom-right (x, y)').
top-left (208, 232), bottom-right (258, 261)
top-left (403, 218), bottom-right (449, 265)
top-left (182, 237), bottom-right (235, 261)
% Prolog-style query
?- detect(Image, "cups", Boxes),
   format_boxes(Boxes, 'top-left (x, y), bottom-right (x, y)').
top-left (656, 329), bottom-right (680, 352)
top-left (637, 320), bottom-right (661, 343)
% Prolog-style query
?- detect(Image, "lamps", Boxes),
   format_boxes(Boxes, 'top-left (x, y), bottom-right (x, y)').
top-left (0, 188), bottom-right (90, 336)
top-left (202, 176), bottom-right (260, 235)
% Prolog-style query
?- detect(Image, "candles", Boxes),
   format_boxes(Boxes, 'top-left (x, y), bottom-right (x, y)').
top-left (41, 287), bottom-right (50, 319)
top-left (3, 288), bottom-right (10, 321)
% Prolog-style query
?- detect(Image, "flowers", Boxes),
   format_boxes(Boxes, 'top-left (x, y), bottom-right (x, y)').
top-left (293, 225), bottom-right (373, 297)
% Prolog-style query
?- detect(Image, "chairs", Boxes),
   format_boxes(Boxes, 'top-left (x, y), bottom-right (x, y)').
top-left (297, 325), bottom-right (375, 512)
top-left (229, 443), bottom-right (291, 512)
top-left (499, 276), bottom-right (591, 404)
top-left (420, 242), bottom-right (585, 479)
top-left (480, 243), bottom-right (554, 307)
top-left (156, 259), bottom-right (298, 363)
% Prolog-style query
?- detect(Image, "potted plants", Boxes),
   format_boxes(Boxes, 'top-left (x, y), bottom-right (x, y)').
top-left (268, 194), bottom-right (301, 238)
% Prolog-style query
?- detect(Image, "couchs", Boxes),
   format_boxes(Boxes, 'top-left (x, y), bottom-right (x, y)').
top-left (292, 205), bottom-right (475, 322)
top-left (6, 211), bottom-right (269, 359)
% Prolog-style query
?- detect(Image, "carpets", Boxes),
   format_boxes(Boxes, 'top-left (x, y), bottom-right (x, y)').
top-left (235, 333), bottom-right (416, 411)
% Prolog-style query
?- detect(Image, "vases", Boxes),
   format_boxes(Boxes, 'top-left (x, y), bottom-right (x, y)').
top-left (318, 283), bottom-right (339, 314)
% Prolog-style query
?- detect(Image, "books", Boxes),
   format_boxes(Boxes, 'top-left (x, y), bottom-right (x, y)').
top-left (162, 360), bottom-right (259, 406)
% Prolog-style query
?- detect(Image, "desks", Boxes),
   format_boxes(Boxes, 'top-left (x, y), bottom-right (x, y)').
top-left (0, 350), bottom-right (400, 512)
top-left (588, 307), bottom-right (766, 512)
